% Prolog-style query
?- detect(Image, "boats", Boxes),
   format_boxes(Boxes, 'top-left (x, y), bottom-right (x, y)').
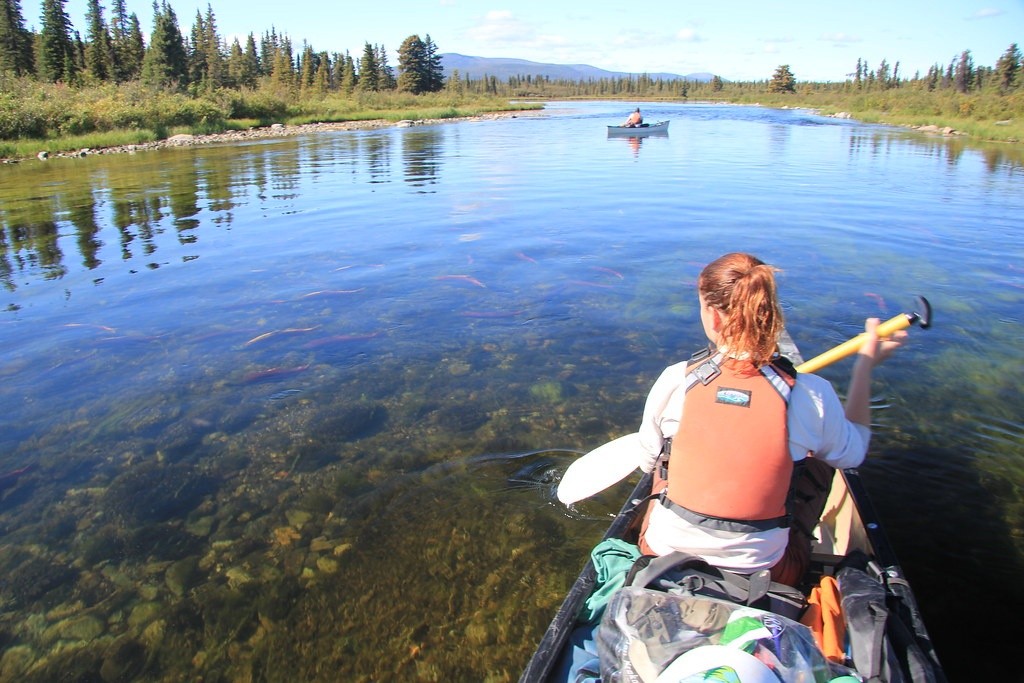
top-left (608, 131), bottom-right (669, 139)
top-left (606, 120), bottom-right (670, 132)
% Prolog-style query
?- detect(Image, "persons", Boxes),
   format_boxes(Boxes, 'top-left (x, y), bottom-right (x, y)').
top-left (626, 107), bottom-right (642, 126)
top-left (636, 253), bottom-right (910, 576)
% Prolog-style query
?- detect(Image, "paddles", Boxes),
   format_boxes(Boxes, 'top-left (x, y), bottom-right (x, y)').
top-left (557, 300), bottom-right (932, 507)
